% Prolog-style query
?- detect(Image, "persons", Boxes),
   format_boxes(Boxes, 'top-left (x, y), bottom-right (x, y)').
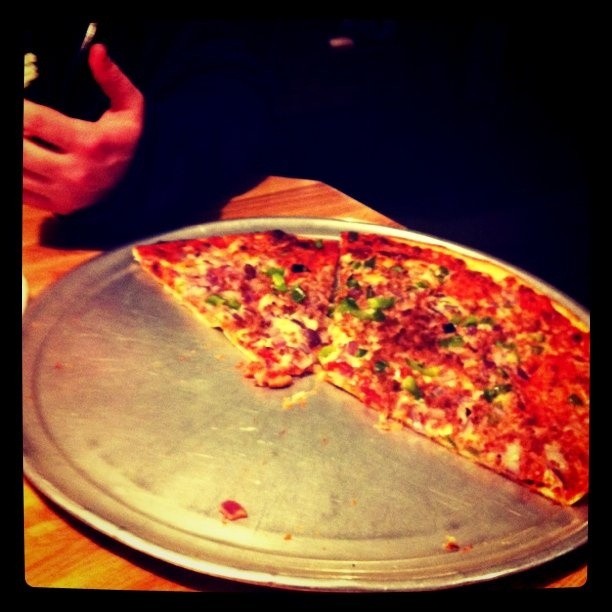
top-left (23, 15), bottom-right (589, 306)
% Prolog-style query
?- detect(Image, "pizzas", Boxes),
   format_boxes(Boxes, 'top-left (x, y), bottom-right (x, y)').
top-left (132, 229), bottom-right (590, 505)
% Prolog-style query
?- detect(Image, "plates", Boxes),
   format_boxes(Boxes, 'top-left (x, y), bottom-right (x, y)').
top-left (21, 215), bottom-right (587, 590)
